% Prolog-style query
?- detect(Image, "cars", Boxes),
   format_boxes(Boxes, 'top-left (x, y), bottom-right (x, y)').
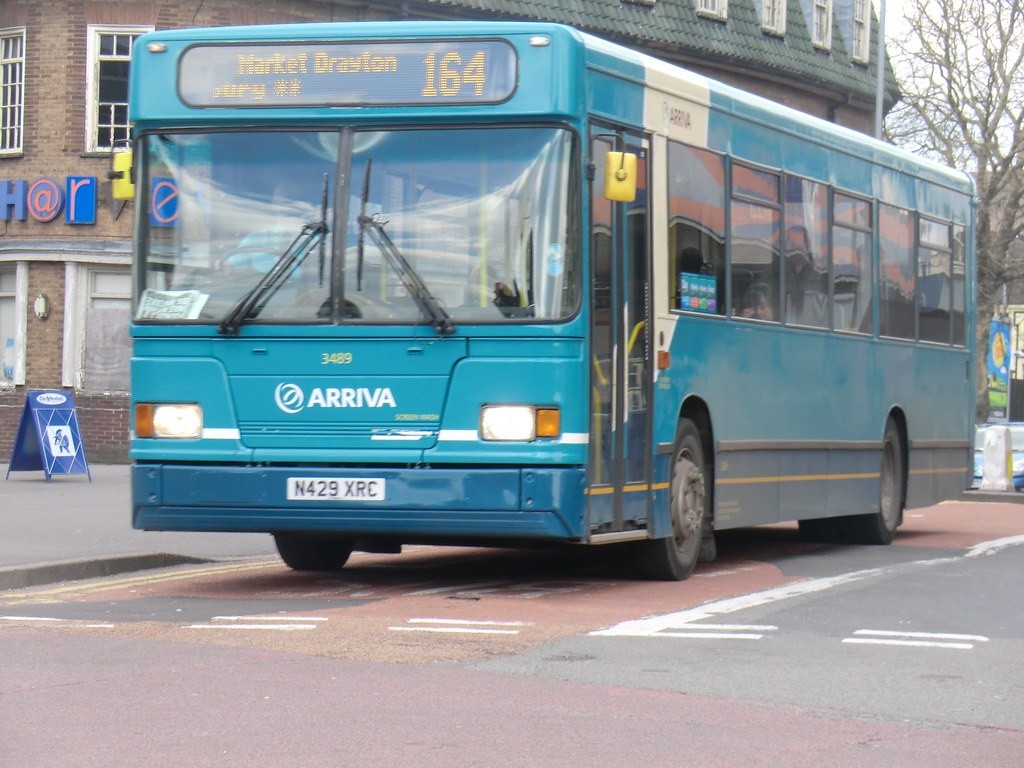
top-left (974, 422), bottom-right (1024, 488)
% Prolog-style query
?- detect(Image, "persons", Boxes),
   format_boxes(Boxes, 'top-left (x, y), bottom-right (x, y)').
top-left (217, 183), bottom-right (332, 281)
top-left (741, 288), bottom-right (773, 321)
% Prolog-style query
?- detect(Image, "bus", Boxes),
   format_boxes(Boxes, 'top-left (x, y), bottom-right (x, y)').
top-left (107, 20), bottom-right (976, 581)
